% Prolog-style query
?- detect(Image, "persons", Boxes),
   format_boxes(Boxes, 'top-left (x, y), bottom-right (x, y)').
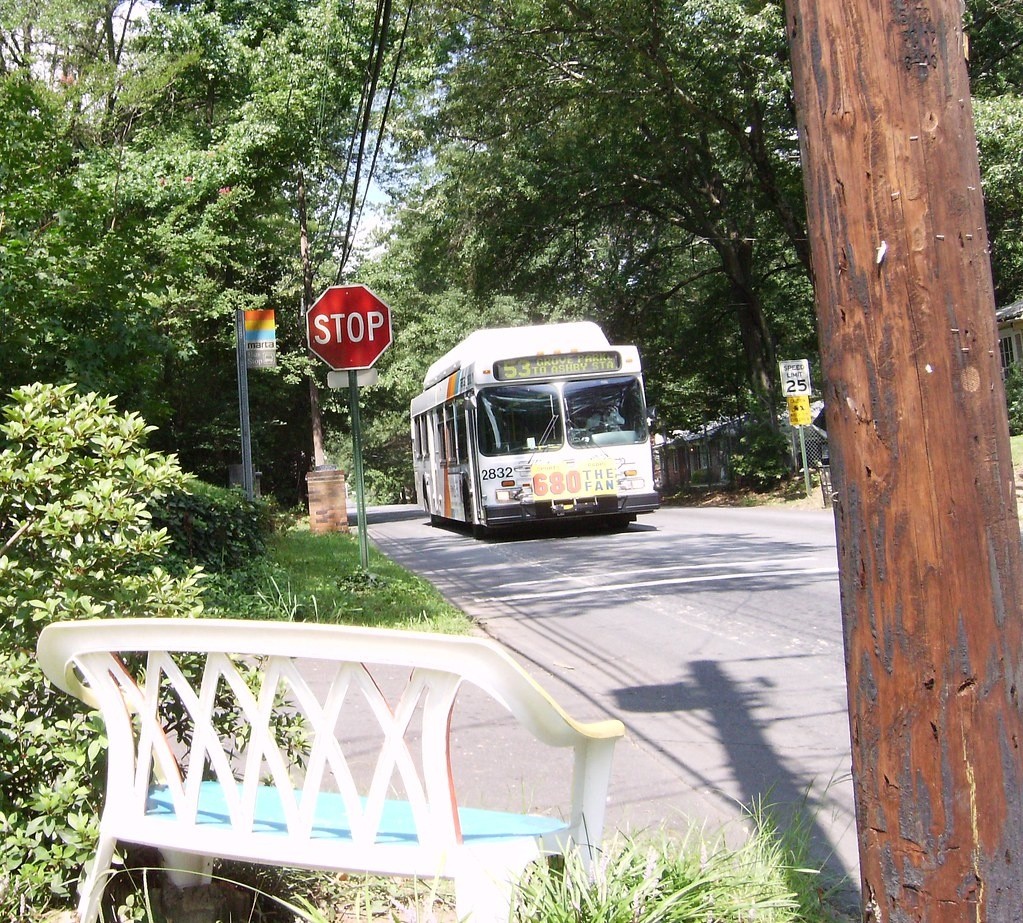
top-left (584, 406), bottom-right (625, 432)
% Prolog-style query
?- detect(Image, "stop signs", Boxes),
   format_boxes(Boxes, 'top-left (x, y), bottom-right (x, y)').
top-left (305, 284), bottom-right (393, 372)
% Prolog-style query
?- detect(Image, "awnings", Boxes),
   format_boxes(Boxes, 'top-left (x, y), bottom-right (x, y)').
top-left (651, 415), bottom-right (747, 449)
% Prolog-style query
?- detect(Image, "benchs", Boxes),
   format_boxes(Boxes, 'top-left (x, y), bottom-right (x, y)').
top-left (36, 617), bottom-right (627, 923)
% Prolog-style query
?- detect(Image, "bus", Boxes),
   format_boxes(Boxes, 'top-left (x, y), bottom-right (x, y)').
top-left (409, 321), bottom-right (661, 540)
